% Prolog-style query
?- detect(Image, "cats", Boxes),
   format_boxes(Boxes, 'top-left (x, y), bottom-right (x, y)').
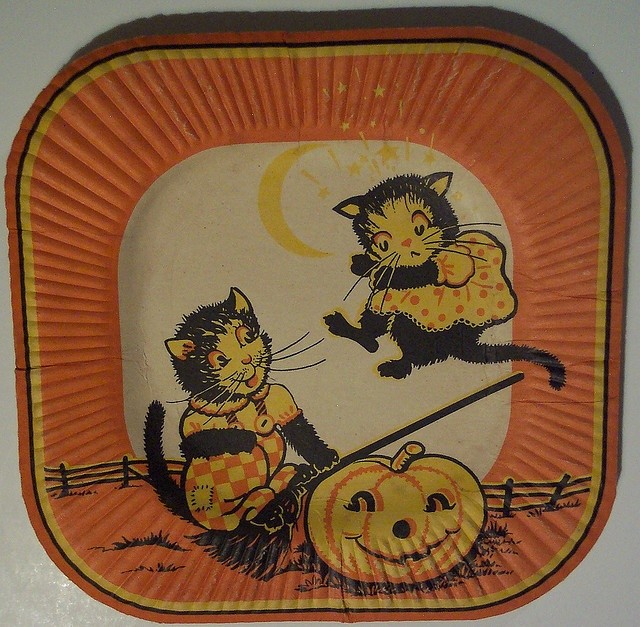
top-left (143, 285), bottom-right (340, 530)
top-left (324, 170), bottom-right (567, 392)
